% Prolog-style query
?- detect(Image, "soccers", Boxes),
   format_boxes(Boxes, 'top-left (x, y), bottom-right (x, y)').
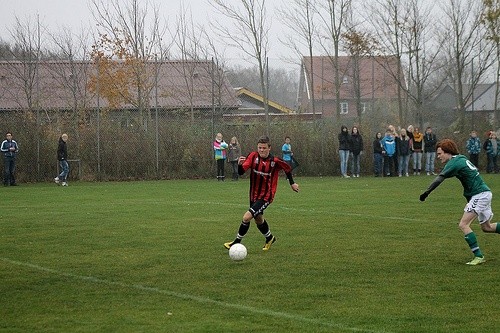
top-left (229, 243), bottom-right (247, 260)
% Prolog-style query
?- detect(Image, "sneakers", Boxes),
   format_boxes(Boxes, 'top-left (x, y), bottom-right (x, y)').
top-left (262, 235), bottom-right (277, 250)
top-left (464, 256), bottom-right (487, 266)
top-left (223, 241), bottom-right (234, 249)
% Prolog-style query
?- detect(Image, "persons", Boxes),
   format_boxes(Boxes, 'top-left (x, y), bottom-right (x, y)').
top-left (0, 132), bottom-right (19, 186)
top-left (423, 127), bottom-right (437, 175)
top-left (227, 136), bottom-right (241, 181)
top-left (412, 128), bottom-right (424, 175)
top-left (419, 139), bottom-right (500, 265)
top-left (282, 137), bottom-right (294, 180)
top-left (55, 134), bottom-right (70, 187)
top-left (373, 132), bottom-right (385, 177)
top-left (213, 133), bottom-right (228, 181)
top-left (380, 126), bottom-right (410, 177)
top-left (224, 136), bottom-right (299, 250)
top-left (465, 131), bottom-right (482, 171)
top-left (351, 126), bottom-right (363, 177)
top-left (338, 125), bottom-right (352, 177)
top-left (406, 126), bottom-right (414, 156)
top-left (484, 132), bottom-right (500, 174)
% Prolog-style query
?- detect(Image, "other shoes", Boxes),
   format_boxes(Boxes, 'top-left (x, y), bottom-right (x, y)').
top-left (54, 177), bottom-right (59, 184)
top-left (404, 173), bottom-right (409, 177)
top-left (61, 181), bottom-right (69, 186)
top-left (426, 172), bottom-right (430, 176)
top-left (340, 174), bottom-right (350, 178)
top-left (412, 171), bottom-right (420, 176)
top-left (398, 174), bottom-right (402, 178)
top-left (352, 174), bottom-right (360, 178)
top-left (431, 172), bottom-right (437, 175)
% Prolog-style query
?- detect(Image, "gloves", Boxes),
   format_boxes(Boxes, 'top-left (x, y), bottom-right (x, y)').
top-left (420, 191), bottom-right (428, 201)
